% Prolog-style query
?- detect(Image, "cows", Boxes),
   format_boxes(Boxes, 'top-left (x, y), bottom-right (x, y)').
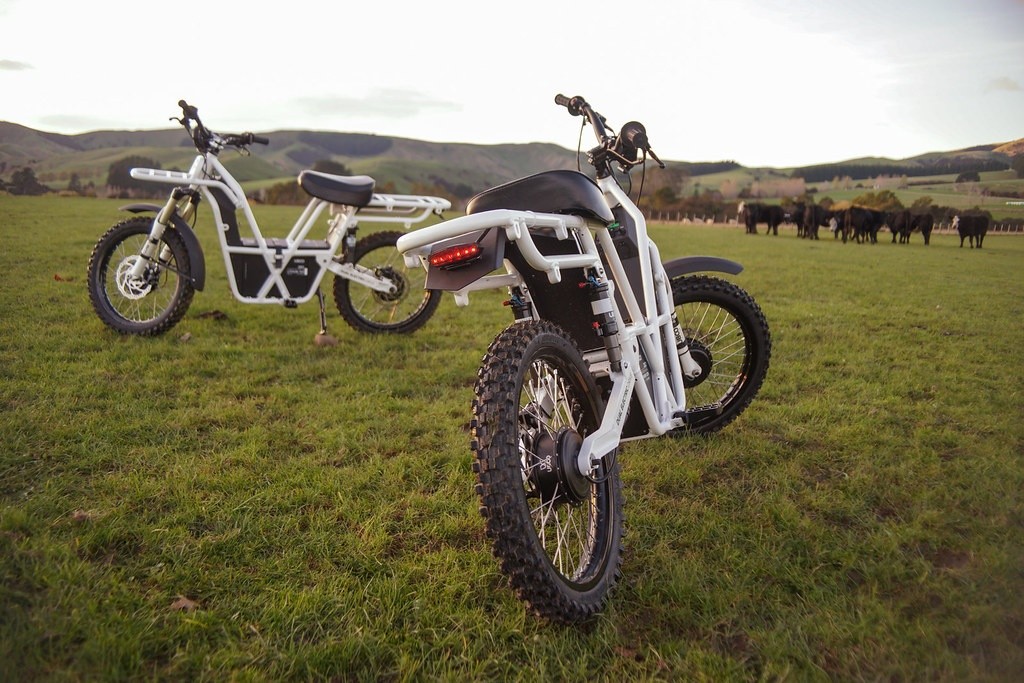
top-left (949, 215), bottom-right (989, 249)
top-left (737, 200), bottom-right (785, 235)
top-left (887, 209), bottom-right (911, 243)
top-left (793, 201), bottom-right (888, 244)
top-left (903, 214), bottom-right (934, 244)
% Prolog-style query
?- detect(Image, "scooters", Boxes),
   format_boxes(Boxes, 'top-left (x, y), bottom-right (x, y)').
top-left (396, 93), bottom-right (772, 625)
top-left (86, 100), bottom-right (452, 337)
